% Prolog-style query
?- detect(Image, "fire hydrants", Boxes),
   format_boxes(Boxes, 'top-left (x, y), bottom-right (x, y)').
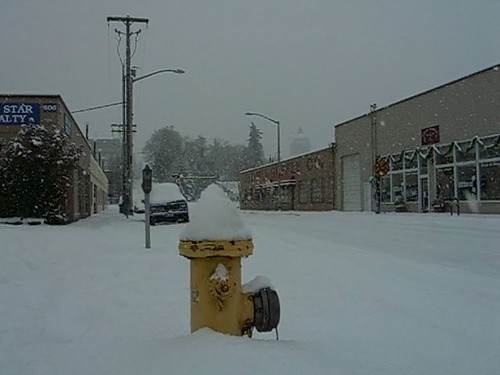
top-left (179, 239), bottom-right (280, 337)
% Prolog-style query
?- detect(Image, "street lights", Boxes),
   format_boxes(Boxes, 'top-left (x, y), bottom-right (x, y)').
top-left (245, 112), bottom-right (281, 208)
top-left (124, 68), bottom-right (185, 213)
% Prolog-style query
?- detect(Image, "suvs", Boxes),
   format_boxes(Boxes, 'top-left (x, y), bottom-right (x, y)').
top-left (150, 182), bottom-right (188, 224)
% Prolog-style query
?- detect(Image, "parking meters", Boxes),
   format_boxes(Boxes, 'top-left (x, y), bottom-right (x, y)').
top-left (142, 164), bottom-right (152, 248)
